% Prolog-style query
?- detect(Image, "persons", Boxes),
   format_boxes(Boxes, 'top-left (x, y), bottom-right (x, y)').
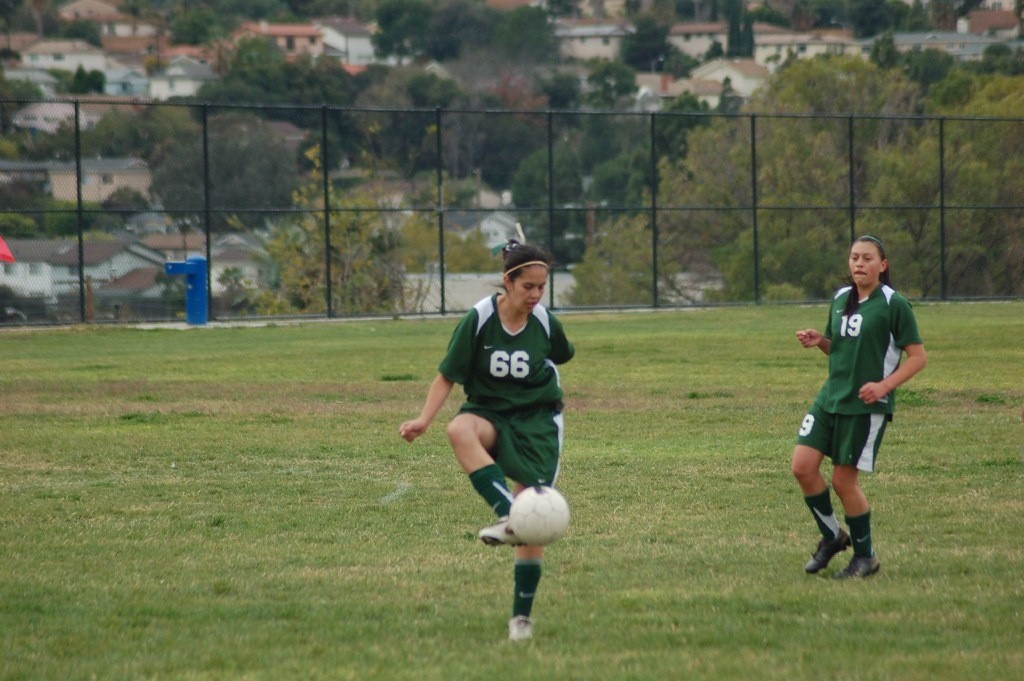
top-left (398, 238), bottom-right (575, 642)
top-left (792, 236), bottom-right (926, 581)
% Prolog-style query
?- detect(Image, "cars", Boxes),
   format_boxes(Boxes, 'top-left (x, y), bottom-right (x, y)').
top-left (0, 269), bottom-right (59, 327)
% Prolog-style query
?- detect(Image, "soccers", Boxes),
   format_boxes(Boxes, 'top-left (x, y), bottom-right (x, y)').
top-left (509, 486), bottom-right (571, 547)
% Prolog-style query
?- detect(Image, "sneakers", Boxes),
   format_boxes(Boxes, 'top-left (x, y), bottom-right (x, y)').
top-left (805, 527), bottom-right (851, 573)
top-left (479, 515), bottom-right (528, 546)
top-left (834, 551), bottom-right (880, 580)
top-left (509, 615), bottom-right (533, 640)
